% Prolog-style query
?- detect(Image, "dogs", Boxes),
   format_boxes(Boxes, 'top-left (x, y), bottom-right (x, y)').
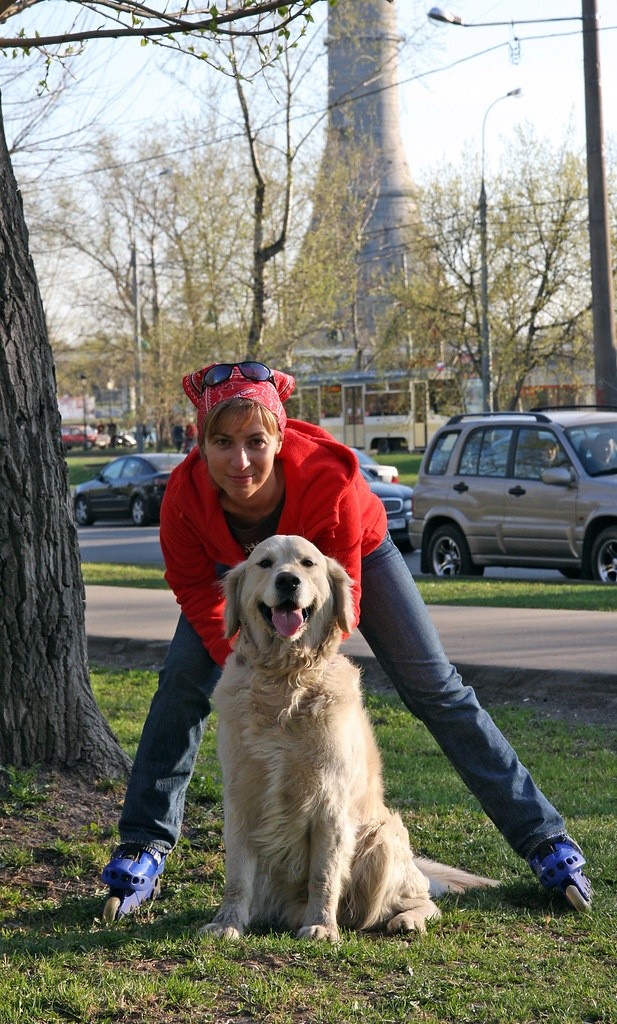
top-left (198, 535), bottom-right (501, 942)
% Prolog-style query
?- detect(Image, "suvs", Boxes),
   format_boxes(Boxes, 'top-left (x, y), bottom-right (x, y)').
top-left (405, 404), bottom-right (617, 585)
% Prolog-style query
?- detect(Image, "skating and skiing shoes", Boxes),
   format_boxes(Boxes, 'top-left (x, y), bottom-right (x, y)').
top-left (97, 837), bottom-right (167, 920)
top-left (523, 836), bottom-right (597, 917)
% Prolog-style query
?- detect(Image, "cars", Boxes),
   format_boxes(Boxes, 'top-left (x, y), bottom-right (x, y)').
top-left (61, 424), bottom-right (97, 450)
top-left (357, 466), bottom-right (414, 554)
top-left (349, 446), bottom-right (401, 484)
top-left (73, 452), bottom-right (192, 528)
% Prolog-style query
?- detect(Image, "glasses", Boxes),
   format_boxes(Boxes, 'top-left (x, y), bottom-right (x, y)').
top-left (197, 361), bottom-right (279, 393)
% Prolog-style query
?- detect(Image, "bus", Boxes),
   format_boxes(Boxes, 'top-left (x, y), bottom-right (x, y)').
top-left (291, 368), bottom-right (471, 455)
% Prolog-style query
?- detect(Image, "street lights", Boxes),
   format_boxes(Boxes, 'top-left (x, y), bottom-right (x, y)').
top-left (477, 86), bottom-right (524, 415)
top-left (131, 167), bottom-right (178, 451)
top-left (427, 5), bottom-right (617, 411)
top-left (80, 374), bottom-right (90, 451)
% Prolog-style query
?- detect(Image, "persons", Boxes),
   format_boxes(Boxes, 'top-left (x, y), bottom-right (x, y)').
top-left (532, 440), bottom-right (563, 477)
top-left (99, 360), bottom-right (596, 922)
top-left (592, 435), bottom-right (617, 469)
